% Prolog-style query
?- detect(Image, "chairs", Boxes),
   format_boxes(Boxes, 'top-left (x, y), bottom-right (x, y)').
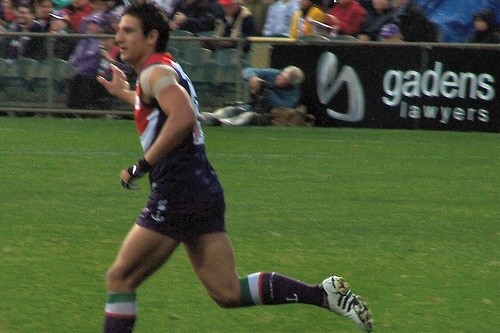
top-left (0, 31), bottom-right (254, 103)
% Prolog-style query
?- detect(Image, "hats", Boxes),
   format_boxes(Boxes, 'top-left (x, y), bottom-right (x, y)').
top-left (380, 23), bottom-right (398, 37)
top-left (82, 11), bottom-right (107, 26)
top-left (49, 9), bottom-right (69, 22)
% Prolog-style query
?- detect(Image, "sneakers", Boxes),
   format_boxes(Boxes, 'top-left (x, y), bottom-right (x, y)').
top-left (323, 275), bottom-right (374, 332)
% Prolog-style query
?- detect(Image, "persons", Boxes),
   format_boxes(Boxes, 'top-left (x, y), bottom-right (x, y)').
top-left (93, 0), bottom-right (372, 333)
top-left (0, 0), bottom-right (500, 119)
top-left (196, 65), bottom-right (306, 126)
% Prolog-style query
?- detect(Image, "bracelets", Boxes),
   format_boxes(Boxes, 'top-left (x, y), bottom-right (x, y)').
top-left (128, 157), bottom-right (152, 180)
